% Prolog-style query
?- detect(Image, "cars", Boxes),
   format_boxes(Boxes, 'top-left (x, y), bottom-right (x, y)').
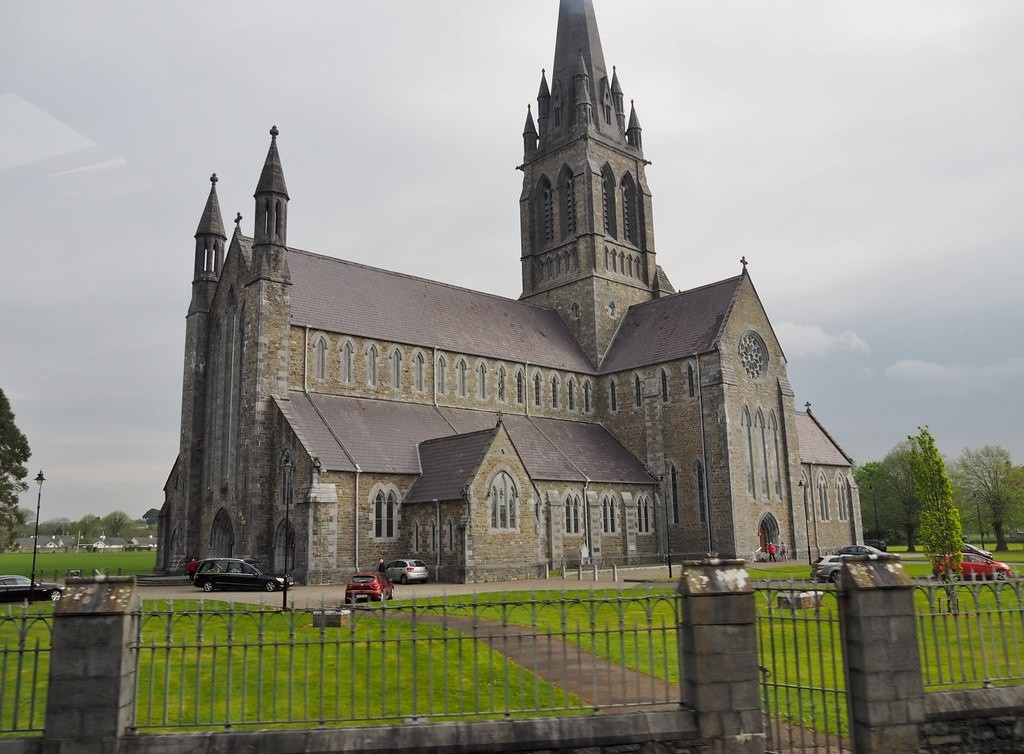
top-left (835, 545), bottom-right (901, 561)
top-left (385, 559), bottom-right (429, 585)
top-left (932, 553), bottom-right (1012, 584)
top-left (345, 572), bottom-right (395, 604)
top-left (810, 554), bottom-right (858, 583)
top-left (0, 574), bottom-right (66, 602)
top-left (961, 544), bottom-right (993, 560)
top-left (194, 557), bottom-right (294, 593)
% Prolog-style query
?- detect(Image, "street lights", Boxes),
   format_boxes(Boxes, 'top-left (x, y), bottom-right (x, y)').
top-left (281, 451), bottom-right (293, 612)
top-left (659, 473), bottom-right (672, 579)
top-left (868, 484), bottom-right (880, 540)
top-left (27, 469), bottom-right (47, 606)
top-left (798, 480), bottom-right (811, 565)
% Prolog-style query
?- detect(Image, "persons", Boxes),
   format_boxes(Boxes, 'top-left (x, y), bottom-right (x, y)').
top-left (769, 542), bottom-right (776, 562)
top-left (379, 558), bottom-right (386, 575)
top-left (780, 542), bottom-right (788, 562)
top-left (207, 562), bottom-right (216, 573)
top-left (188, 558), bottom-right (198, 584)
